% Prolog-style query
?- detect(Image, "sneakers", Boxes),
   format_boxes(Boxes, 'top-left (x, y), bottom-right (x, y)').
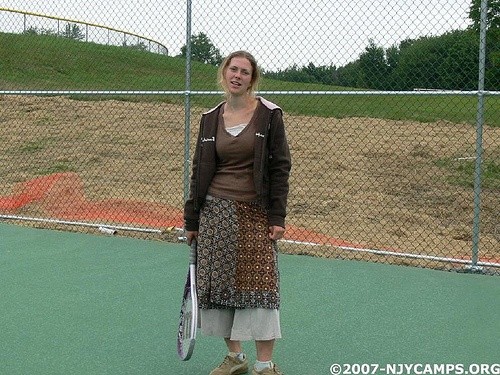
top-left (252, 363), bottom-right (283, 375)
top-left (208, 353), bottom-right (248, 375)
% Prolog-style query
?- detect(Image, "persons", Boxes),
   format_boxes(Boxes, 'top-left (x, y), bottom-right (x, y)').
top-left (184, 51), bottom-right (291, 375)
